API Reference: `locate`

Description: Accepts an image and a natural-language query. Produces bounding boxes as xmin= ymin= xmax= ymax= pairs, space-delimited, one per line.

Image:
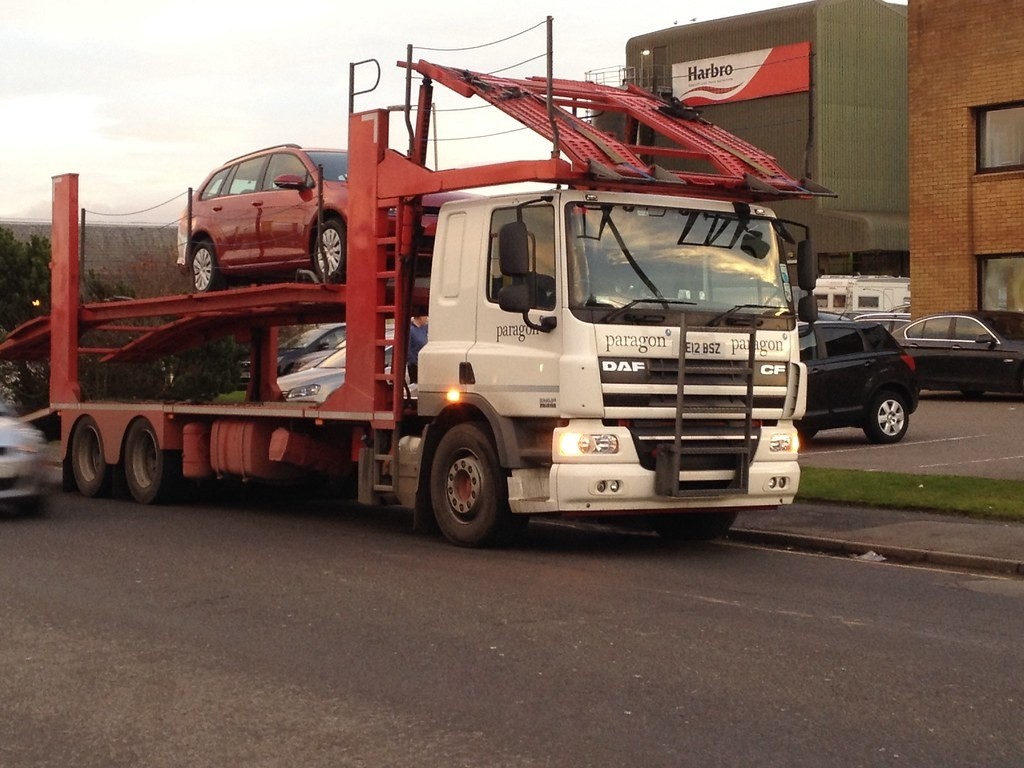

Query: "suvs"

xmin=796 ymin=321 xmax=923 ymax=447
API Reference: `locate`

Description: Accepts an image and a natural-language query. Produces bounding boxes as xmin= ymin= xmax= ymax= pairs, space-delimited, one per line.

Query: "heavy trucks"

xmin=1 ymin=14 xmax=839 ymax=552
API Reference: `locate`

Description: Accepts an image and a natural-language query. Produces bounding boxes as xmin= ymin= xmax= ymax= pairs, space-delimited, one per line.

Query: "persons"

xmin=407 ymin=316 xmax=429 ymax=384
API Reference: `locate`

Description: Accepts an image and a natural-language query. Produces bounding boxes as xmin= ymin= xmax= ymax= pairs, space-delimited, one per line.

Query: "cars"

xmin=888 ymin=310 xmax=1024 ymax=400
xmin=1 ymin=418 xmax=44 ymax=519
xmin=816 ymin=309 xmax=912 ymax=334
